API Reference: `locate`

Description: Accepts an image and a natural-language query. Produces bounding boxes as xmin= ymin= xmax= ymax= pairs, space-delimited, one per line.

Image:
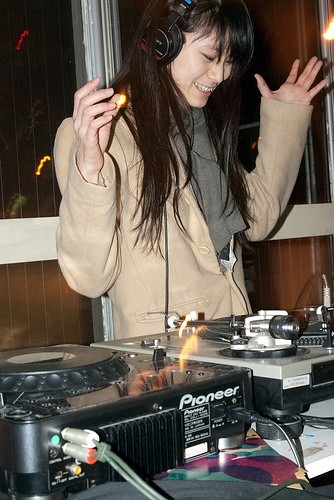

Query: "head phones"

xmin=140 ymin=0 xmax=200 ymax=68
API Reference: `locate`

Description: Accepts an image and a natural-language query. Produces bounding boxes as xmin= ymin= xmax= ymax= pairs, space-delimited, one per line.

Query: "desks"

xmin=250 ymin=398 xmax=334 ymax=479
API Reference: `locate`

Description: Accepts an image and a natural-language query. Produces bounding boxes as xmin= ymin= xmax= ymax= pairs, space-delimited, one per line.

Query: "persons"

xmin=52 ymin=0 xmax=331 ymax=337
